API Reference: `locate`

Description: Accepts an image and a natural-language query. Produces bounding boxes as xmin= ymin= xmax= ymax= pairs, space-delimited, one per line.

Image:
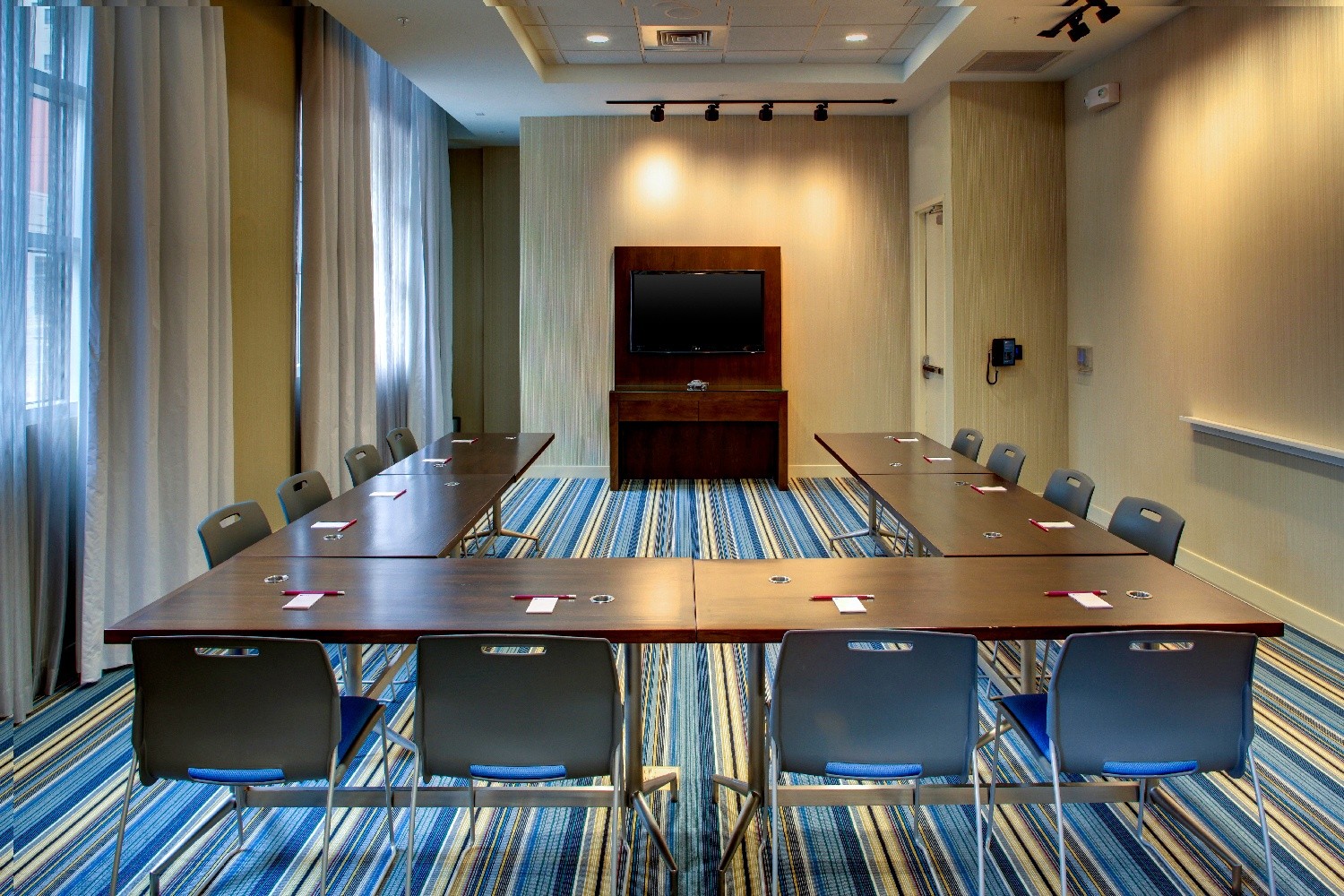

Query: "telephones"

xmin=992 ymin=338 xmax=1015 ymax=366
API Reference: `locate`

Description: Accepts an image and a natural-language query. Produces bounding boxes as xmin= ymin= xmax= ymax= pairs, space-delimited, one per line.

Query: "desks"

xmin=857 ymin=472 xmax=1150 ymax=558
xmin=237 ymin=475 xmax=542 ymax=558
xmin=103 ymin=549 xmax=694 ymax=896
xmin=691 ymin=555 xmax=1284 ymax=892
xmin=608 ymin=389 xmax=789 ymax=491
xmin=811 ymin=431 xmax=999 ymax=551
xmin=375 ymin=432 xmax=556 ymax=548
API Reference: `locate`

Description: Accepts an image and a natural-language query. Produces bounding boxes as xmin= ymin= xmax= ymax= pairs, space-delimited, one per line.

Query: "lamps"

xmin=1067 ymin=15 xmax=1090 ymax=43
xmin=650 ymin=103 xmax=665 ymax=122
xmin=1089 ymin=0 xmax=1120 ymax=23
xmin=814 ymin=102 xmax=828 ymax=121
xmin=759 ymin=103 xmax=773 ymax=121
xmin=705 ymin=104 xmax=719 ymax=121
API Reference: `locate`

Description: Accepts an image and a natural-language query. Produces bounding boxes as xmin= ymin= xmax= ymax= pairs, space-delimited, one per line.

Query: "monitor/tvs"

xmin=630 ymin=269 xmax=766 ymax=355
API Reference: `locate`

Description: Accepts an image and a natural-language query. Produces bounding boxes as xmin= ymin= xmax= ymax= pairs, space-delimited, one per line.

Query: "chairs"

xmin=109 ymin=426 xmax=1277 ymax=895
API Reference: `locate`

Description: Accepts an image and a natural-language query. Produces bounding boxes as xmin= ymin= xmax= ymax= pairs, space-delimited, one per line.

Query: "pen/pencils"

xmin=510 ymin=594 xmax=577 ymax=599
xmin=970 ymin=485 xmax=986 ymax=495
xmin=1028 ymin=519 xmax=1048 ymax=532
xmin=393 ymin=489 xmax=407 ymax=500
xmin=443 ymin=457 xmax=452 ymax=464
xmin=471 ymin=438 xmax=479 ymax=444
xmin=923 ymin=455 xmax=933 ymax=464
xmin=893 ymin=437 xmax=901 ymax=443
xmin=338 ymin=518 xmax=357 ymax=532
xmin=280 ymin=589 xmax=346 ymax=596
xmin=1043 ymin=590 xmax=1108 ymax=596
xmin=810 ymin=594 xmax=875 ymax=600
